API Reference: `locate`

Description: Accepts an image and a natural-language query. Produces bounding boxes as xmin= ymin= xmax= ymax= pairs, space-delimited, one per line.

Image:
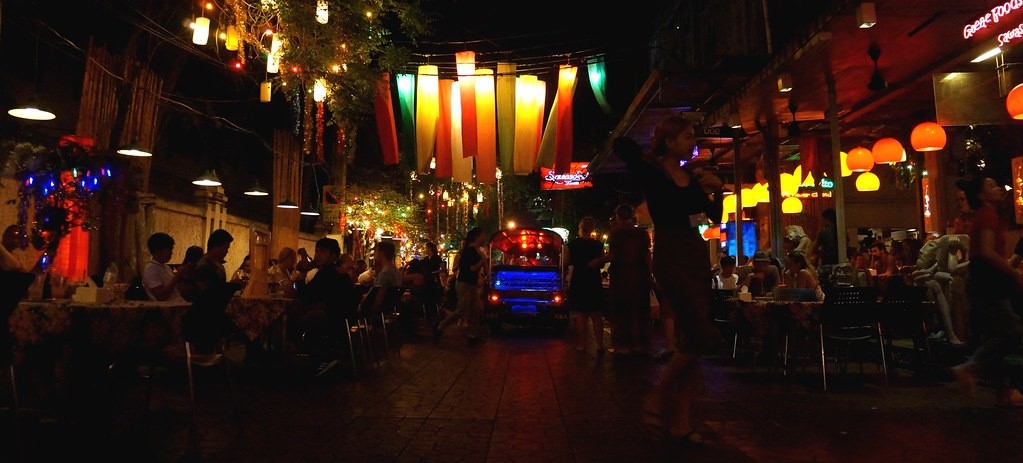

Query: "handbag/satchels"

xmin=648 ymin=288 xmax=662 ymax=322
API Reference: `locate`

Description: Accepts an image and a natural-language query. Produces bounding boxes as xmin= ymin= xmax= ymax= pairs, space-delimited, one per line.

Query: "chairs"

xmin=821 ymin=286 xmax=890 ymax=391
xmin=372 ymin=286 xmax=405 ymax=362
xmin=358 ymin=285 xmax=382 ymax=369
xmin=876 ymin=284 xmax=937 ymax=384
xmin=320 ymin=284 xmax=371 ymax=380
xmin=711 ymin=289 xmax=736 ymax=328
xmin=138 ymin=280 xmax=241 ymax=457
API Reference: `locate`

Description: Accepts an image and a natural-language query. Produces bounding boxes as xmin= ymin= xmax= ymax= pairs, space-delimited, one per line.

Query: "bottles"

xmin=103 ymin=262 xmax=119 ymax=289
xmin=268 ymin=276 xmax=285 ymax=296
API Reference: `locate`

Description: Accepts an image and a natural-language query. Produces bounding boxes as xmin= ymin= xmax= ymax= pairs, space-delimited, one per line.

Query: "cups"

xmin=766 ymin=290 xmax=773 ymax=301
xmin=28 ymin=274 xmax=45 ymax=300
xmin=50 ymin=274 xmax=69 ymax=301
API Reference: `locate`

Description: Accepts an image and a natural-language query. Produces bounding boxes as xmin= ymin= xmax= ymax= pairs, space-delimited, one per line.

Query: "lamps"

xmin=267 ymin=54 xmax=279 ymax=73
xmin=1005 ymin=83 xmax=1023 ymax=121
xmin=374 ymin=48 xmax=610 ymax=185
xmin=300 ymin=202 xmax=321 ymax=216
xmin=243 ymin=178 xmax=269 ymax=196
xmin=260 ymin=73 xmax=272 ymax=103
xmin=191 ymin=168 xmax=222 ymax=187
xmin=6 ymin=26 xmax=56 ymax=121
xmin=313 ymin=77 xmax=326 ymax=103
xmin=315 ymin=0 xmax=328 ymax=24
xmin=192 ymin=0 xmax=210 ymax=46
xmin=271 ymin=24 xmax=284 ymax=56
xmin=846 ymin=147 xmax=874 ymax=172
xmin=225 ymin=25 xmax=239 ymax=51
xmin=855 ymin=171 xmax=880 ymax=191
xmin=116 ymin=93 xmax=153 ymax=158
xmin=872 ymin=138 xmax=906 ymax=164
xmin=910 ymin=122 xmax=947 ymax=152
xmin=708 ymin=151 xmax=853 ymax=224
xmin=776 ymin=73 xmax=793 ymax=93
xmin=276 ymin=197 xmax=299 ymax=209
xmin=855 ymin=2 xmax=877 ymax=29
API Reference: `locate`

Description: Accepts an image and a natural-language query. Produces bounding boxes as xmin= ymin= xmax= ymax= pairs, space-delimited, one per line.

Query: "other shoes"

xmin=467 ymin=335 xmax=487 ymax=344
xmin=430 ymin=321 xmax=442 ymax=339
xmin=313 ymin=355 xmax=337 ymax=377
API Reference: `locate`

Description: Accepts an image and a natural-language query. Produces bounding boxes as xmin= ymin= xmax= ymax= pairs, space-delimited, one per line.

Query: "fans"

xmin=866 ymin=42 xmax=902 ymax=92
xmin=705 ymin=147 xmax=733 ymax=169
xmin=736 ymin=122 xmax=760 ymax=143
xmin=785 ymin=99 xmax=831 ymax=140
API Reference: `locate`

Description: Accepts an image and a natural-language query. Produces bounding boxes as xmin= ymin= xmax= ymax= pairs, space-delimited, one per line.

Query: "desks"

xmin=227 ymin=294 xmax=299 ymax=396
xmin=729 ymin=300 xmax=936 ymax=384
xmin=7 ymin=298 xmax=193 ymax=463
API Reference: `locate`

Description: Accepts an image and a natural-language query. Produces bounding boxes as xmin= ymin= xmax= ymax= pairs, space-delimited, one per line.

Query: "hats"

xmin=754 ymin=251 xmax=770 ymax=263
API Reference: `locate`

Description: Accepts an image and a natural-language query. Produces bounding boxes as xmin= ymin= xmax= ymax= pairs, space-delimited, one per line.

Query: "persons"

xmin=0 ymin=115 xmax=1023 ymax=463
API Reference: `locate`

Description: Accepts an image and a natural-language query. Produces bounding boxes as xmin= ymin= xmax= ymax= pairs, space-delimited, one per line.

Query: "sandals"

xmin=679 ymin=430 xmax=710 ymax=450
